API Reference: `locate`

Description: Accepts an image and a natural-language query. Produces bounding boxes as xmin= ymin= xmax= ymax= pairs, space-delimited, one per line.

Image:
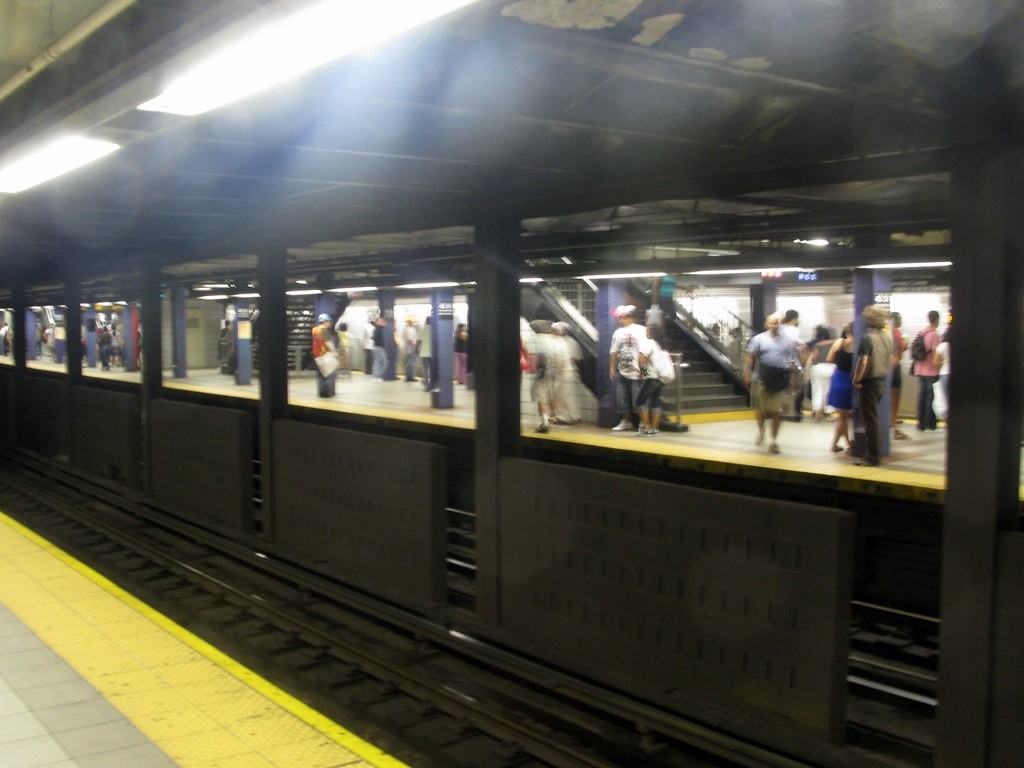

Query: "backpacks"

xmin=650 ymin=339 xmax=675 ymax=384
xmin=911 ymin=330 xmax=934 ymax=362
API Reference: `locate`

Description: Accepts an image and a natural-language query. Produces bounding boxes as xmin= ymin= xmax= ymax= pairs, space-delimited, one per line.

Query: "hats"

xmin=318 ymin=313 xmax=331 ymax=322
xmin=405 ymin=317 xmax=416 ymax=323
xmin=614 ymin=305 xmax=636 ymax=318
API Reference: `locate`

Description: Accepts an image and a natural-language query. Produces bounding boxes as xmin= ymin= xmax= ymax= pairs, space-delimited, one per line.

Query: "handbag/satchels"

xmin=315 ymin=352 xmax=339 ymax=377
xmin=759 ymin=365 xmax=793 ymax=392
xmin=530 ymin=353 xmax=545 ymax=380
xmin=646 ymin=303 xmax=663 ymax=326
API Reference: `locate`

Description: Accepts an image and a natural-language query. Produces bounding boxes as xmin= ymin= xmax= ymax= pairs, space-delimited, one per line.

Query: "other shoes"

xmin=633 ymin=428 xmax=658 ymax=439
xmin=833 ymin=447 xmax=843 ymax=452
xmin=612 ymin=419 xmax=633 ymax=431
xmin=854 ymin=462 xmax=879 ymax=467
xmin=407 ymin=378 xmax=418 ymax=381
xmin=537 ymin=424 xmax=548 ymax=433
xmin=895 ymin=430 xmax=911 ymax=441
xmin=917 ymin=424 xmax=944 ymax=433
xmin=756 ymin=436 xmax=763 ymax=445
xmin=770 ymin=445 xmax=778 ymax=454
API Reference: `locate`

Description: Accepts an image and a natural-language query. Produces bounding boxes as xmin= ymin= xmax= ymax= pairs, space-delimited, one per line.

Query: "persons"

xmin=518 ymin=274 xmax=951 ymax=467
xmin=312 ymin=310 xmax=469 ymax=398
xmin=0 ymin=319 xmax=142 ymax=372
xmin=219 ymin=320 xmax=229 ymax=339
